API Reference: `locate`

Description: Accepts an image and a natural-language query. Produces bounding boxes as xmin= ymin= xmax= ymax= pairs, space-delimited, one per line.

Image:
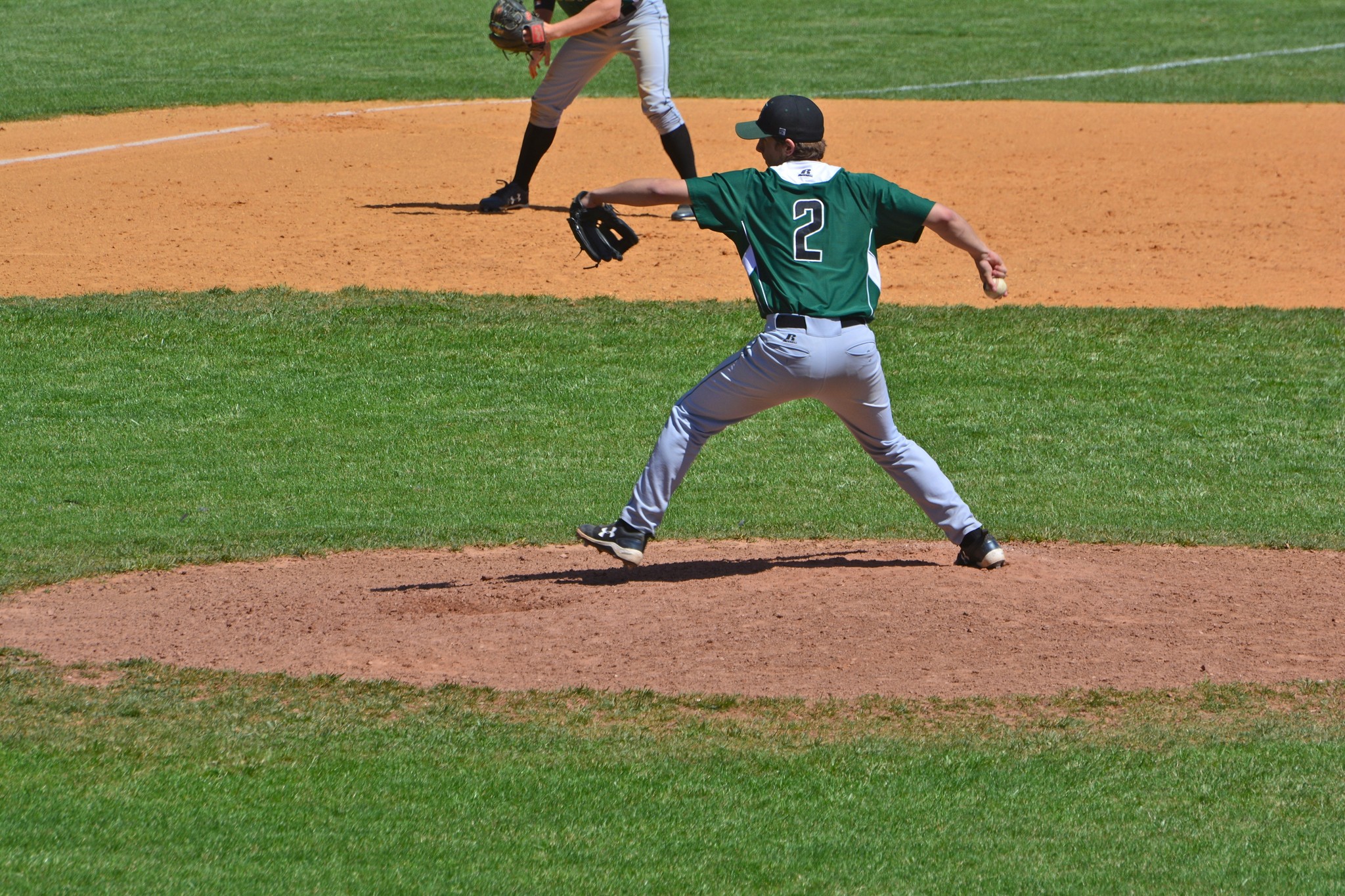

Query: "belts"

xmin=775 ymin=314 xmax=868 ymax=330
xmin=611 ymin=3 xmax=636 ymax=25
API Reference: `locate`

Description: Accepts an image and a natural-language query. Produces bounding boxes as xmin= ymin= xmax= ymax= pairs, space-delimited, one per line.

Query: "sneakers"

xmin=671 ymin=204 xmax=696 ymax=221
xmin=953 ymin=528 xmax=1006 ymax=571
xmin=574 ymin=518 xmax=649 ymax=570
xmin=478 ymin=179 xmax=530 ymax=214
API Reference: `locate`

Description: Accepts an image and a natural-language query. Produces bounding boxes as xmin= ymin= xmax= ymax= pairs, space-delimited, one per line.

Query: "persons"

xmin=477 ymin=0 xmax=695 ymax=221
xmin=575 ymin=94 xmax=1007 ymax=571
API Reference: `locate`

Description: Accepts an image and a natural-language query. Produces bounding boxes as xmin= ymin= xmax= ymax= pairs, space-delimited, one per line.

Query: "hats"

xmin=735 ymin=95 xmax=825 ymax=144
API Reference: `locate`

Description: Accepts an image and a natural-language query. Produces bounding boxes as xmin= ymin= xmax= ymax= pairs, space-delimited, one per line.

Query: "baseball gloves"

xmin=489 ymin=0 xmax=545 ymax=53
xmin=567 ymin=191 xmax=639 ymax=263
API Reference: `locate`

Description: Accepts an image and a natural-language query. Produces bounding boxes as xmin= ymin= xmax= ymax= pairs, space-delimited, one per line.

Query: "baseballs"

xmin=983 ymin=276 xmax=1007 ymax=299
